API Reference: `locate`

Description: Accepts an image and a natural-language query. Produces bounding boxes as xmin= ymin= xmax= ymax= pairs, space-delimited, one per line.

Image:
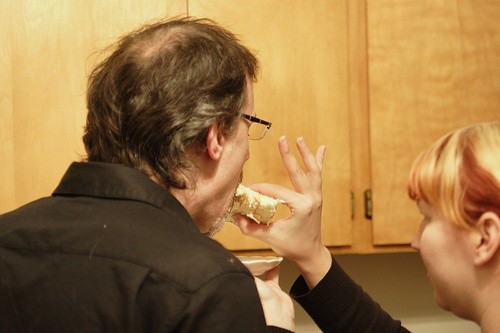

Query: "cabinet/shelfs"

xmin=1 ymin=1 xmax=500 ymax=258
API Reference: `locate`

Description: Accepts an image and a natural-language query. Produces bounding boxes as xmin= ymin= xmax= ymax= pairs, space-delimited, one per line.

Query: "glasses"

xmin=236 ymin=112 xmax=272 ymax=141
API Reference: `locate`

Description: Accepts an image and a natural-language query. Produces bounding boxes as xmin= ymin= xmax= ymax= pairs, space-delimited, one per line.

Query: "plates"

xmin=235 ymin=254 xmax=282 ymax=278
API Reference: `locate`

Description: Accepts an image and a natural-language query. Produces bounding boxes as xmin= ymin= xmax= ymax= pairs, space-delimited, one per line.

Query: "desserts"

xmin=224 ymin=184 xmax=283 ymax=226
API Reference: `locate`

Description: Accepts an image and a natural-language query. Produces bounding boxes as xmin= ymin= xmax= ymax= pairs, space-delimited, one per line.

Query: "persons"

xmin=249 ymin=121 xmax=500 ymax=333
xmin=0 ymin=16 xmax=273 ymax=333
